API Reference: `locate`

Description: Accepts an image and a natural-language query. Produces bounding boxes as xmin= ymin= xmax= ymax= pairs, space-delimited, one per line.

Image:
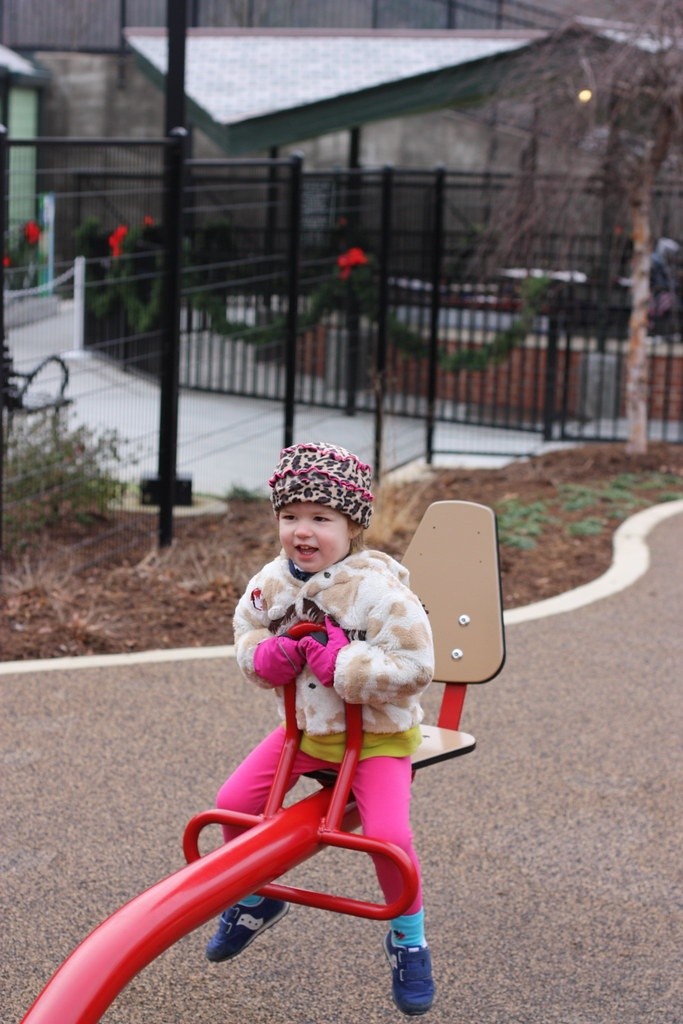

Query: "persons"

xmin=609 ymin=237 xmax=681 ymax=322
xmin=205 ymin=441 xmax=471 ymax=1017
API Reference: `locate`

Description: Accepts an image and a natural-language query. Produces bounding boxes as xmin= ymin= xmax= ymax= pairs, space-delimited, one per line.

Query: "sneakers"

xmin=206 ymin=894 xmax=289 ymax=963
xmin=383 ymin=930 xmax=435 ymax=1015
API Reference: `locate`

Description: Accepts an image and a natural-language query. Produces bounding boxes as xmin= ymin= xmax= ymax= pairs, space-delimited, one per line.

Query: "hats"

xmin=269 ymin=441 xmax=373 ymax=530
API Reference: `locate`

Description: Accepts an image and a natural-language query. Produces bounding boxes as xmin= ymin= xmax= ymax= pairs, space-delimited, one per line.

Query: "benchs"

xmin=0 ymin=331 xmax=72 ymax=449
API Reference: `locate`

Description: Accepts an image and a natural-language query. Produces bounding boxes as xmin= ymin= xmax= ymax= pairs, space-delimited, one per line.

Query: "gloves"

xmin=254 ymin=636 xmax=306 ymax=685
xmin=297 ymin=612 xmax=349 ymax=688
